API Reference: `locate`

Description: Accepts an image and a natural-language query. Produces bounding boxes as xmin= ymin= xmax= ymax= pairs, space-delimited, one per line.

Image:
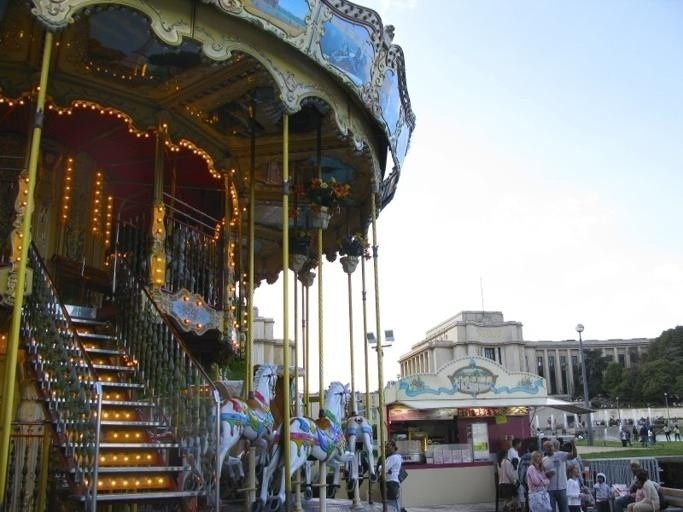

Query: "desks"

xmin=614 ymin=396 xmax=621 ymax=419
xmin=367 ymin=329 xmax=394 ymax=355
xmin=663 ymin=392 xmax=671 ymax=418
xmin=574 ymin=323 xmax=594 ymax=447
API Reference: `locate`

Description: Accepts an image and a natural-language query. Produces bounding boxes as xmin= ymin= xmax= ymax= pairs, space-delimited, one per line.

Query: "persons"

xmin=378 ymin=440 xmax=402 ymax=500
xmin=494 ymin=414 xmax=683 ymax=512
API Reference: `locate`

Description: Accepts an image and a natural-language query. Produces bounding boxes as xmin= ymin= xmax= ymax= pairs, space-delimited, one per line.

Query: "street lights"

xmin=613 ymin=484 xmax=627 ymax=495
xmin=398 ymin=467 xmax=408 ymax=482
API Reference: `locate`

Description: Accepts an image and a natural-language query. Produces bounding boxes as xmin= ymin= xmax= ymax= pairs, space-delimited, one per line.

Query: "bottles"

xmin=597 ymin=473 xmax=606 ymax=482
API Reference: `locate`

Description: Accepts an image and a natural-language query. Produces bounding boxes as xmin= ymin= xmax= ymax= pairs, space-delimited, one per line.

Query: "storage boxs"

xmin=656 ymin=487 xmax=683 ymax=512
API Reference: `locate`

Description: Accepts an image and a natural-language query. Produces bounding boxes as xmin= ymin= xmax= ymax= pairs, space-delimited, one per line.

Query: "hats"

xmin=591 ymin=415 xmax=682 ymax=426
xmin=571 ymin=427 xmax=591 ymax=441
